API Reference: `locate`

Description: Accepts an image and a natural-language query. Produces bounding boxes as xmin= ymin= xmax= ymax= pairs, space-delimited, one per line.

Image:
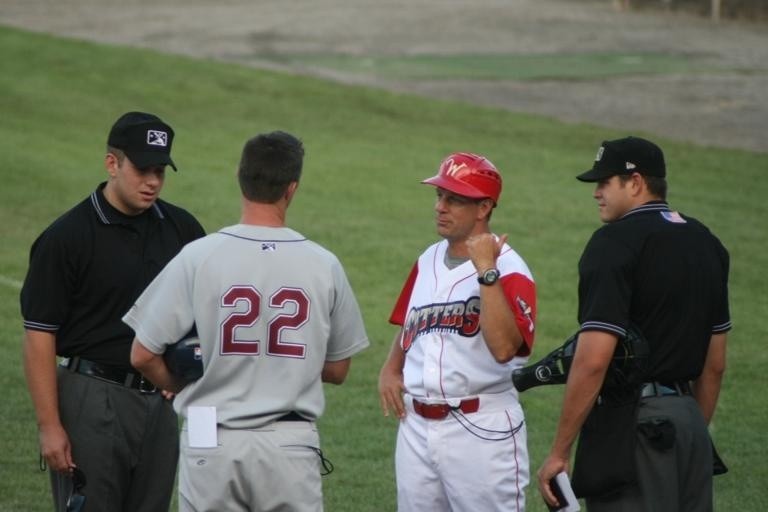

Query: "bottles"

xmin=577 ymin=136 xmax=664 ymax=182
xmin=109 ymin=111 xmax=178 ymax=173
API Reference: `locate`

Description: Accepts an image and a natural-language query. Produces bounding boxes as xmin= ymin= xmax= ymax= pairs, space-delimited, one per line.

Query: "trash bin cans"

xmin=476 ymin=268 xmax=501 ymax=285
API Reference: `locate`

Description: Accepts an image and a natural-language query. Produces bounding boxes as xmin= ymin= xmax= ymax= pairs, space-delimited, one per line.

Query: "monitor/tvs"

xmin=542 ymin=472 xmax=582 ymax=512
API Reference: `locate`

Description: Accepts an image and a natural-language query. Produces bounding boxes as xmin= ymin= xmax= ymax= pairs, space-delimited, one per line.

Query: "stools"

xmin=640 ymin=379 xmax=691 ymax=399
xmin=413 ymin=398 xmax=479 ymax=419
xmin=58 ymin=356 xmax=159 ymax=395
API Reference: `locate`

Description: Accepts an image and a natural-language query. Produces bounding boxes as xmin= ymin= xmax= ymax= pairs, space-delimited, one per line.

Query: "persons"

xmin=534 ymin=132 xmax=735 ymax=512
xmin=118 ymin=128 xmax=371 ymax=511
xmin=18 ymin=109 xmax=207 ymax=512
xmin=376 ymin=150 xmax=541 ymax=512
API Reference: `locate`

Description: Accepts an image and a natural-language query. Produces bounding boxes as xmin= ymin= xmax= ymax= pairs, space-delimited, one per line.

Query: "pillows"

xmin=420 ymin=152 xmax=502 ymax=207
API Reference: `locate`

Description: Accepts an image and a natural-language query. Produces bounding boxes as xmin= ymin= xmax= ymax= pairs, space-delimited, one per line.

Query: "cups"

xmin=65 ymin=466 xmax=87 ymax=511
